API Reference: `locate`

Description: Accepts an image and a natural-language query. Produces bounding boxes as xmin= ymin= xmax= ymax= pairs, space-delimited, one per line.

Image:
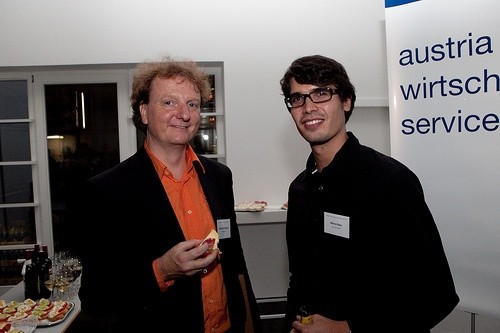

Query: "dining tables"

xmin=0 ymin=276 xmax=85 ymax=333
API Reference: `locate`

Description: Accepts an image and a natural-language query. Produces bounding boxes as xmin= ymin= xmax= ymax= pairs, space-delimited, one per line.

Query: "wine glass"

xmin=44 ymin=250 xmax=83 ymax=301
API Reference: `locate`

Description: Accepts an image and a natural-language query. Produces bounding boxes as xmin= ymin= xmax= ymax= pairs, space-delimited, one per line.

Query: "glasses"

xmin=284 ymin=86 xmax=338 ymax=109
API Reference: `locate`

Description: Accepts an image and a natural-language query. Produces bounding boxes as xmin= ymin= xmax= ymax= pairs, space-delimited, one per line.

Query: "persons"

xmin=71 ymin=62 xmax=264 ymax=333
xmin=281 ymin=55 xmax=460 ymax=333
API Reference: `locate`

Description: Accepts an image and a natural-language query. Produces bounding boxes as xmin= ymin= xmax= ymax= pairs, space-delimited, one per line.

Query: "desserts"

xmin=200 ymin=229 xmax=219 ymax=254
xmin=0 ymin=298 xmax=69 ymax=333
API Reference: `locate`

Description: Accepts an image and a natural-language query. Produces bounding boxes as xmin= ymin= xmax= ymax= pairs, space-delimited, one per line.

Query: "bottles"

xmin=300 ymin=305 xmax=313 ymax=325
xmin=24 ymin=245 xmax=52 ymax=301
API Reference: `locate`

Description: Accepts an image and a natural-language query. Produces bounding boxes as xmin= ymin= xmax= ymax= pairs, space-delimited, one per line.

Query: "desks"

xmin=235 ymin=208 xmax=294 ymax=318
